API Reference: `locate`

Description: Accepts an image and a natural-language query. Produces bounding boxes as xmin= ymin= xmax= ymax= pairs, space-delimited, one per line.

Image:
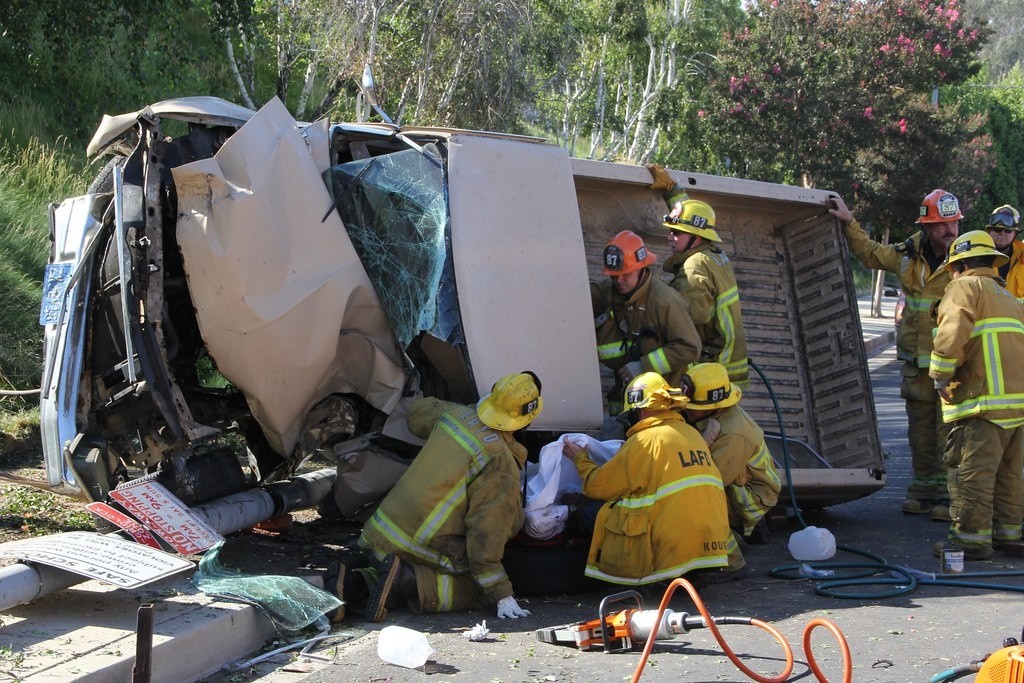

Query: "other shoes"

xmin=696 ymin=566 xmax=746 ymax=584
xmin=669 ymin=568 xmax=702 ymax=596
xmin=933 ymin=541 xmax=992 ymax=561
xmin=599 ymin=583 xmax=629 ymax=601
xmin=901 ymin=497 xmax=934 ymax=513
xmin=991 ymin=537 xmax=1022 ymax=549
xmin=931 ymin=506 xmax=953 ymax=521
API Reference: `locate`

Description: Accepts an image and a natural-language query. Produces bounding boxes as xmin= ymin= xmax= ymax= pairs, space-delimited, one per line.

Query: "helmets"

xmin=478 ymin=371 xmax=542 ymax=432
xmin=943 ymin=230 xmax=1010 ymax=272
xmin=985 ymin=204 xmax=1024 ymax=232
xmin=620 ymin=372 xmax=690 ymax=416
xmin=663 ymin=197 xmax=724 ymax=245
xmin=675 ymin=362 xmax=742 ymax=409
xmin=914 ymin=188 xmax=964 ymax=224
xmin=601 ymin=230 xmax=657 ymax=276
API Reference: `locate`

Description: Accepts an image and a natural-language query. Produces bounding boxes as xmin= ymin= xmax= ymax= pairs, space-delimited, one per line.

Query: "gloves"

xmin=646 ymin=163 xmax=676 ymax=193
xmin=496 ymin=595 xmax=531 ymax=619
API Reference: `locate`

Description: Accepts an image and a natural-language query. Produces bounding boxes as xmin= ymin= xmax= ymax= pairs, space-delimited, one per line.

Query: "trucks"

xmin=37 ymin=96 xmax=891 ymax=538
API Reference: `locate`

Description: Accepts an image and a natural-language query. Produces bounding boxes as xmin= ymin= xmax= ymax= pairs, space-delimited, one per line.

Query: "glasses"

xmin=988 ymin=213 xmax=1017 ymax=226
xmin=670 ymin=228 xmax=689 ymax=235
xmin=992 ymin=228 xmax=1014 ymax=233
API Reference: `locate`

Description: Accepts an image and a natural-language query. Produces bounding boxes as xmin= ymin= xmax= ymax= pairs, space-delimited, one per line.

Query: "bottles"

xmin=799 ymin=563 xmax=834 ymax=576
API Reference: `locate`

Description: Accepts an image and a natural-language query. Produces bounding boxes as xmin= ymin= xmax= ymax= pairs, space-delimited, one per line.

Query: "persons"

xmin=563 ymin=372 xmax=731 ymax=598
xmin=928 ymin=202 xmax=1024 ymax=558
xmin=677 ymin=362 xmax=781 ymax=546
xmin=829 ymin=189 xmax=965 ymax=521
xmin=645 ymin=164 xmax=750 ymax=391
xmin=589 ymin=230 xmax=702 ymax=416
xmin=322 ymin=370 xmax=544 ymax=623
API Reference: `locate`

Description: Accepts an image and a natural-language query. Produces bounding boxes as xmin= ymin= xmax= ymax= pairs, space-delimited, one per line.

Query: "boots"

xmin=322 ymin=560 xmax=371 ymax=624
xmin=366 ymin=553 xmax=417 ymax=623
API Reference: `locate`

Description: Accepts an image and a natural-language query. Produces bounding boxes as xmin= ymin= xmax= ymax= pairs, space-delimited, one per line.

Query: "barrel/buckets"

xmin=378 ymin=626 xmax=434 ymax=669
xmin=941 ymin=549 xmax=964 ymax=574
xmin=785 ymin=525 xmax=836 ymax=561
